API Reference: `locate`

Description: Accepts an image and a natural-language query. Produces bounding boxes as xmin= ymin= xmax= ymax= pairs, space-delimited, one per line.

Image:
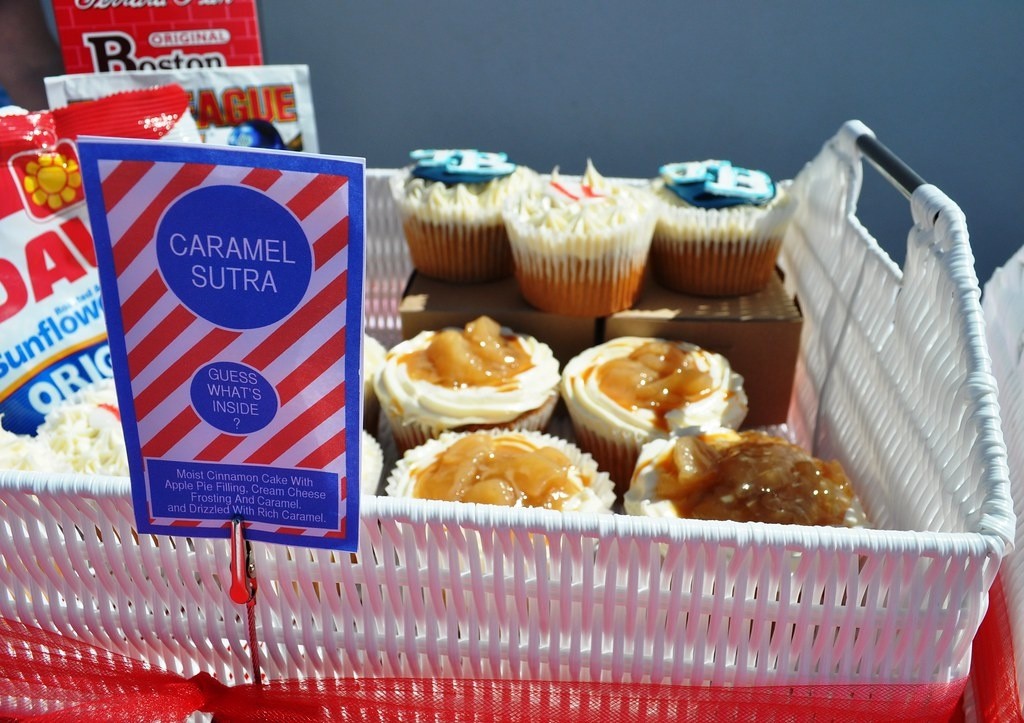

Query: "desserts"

xmin=389 ymin=148 xmax=802 ymax=318
xmin=365 ymin=316 xmax=868 ymax=535
xmin=0 ymin=379 xmax=131 ymax=483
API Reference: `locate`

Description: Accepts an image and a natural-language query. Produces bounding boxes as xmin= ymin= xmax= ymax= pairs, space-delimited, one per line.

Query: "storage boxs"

xmin=396 ymin=268 xmax=805 ymax=428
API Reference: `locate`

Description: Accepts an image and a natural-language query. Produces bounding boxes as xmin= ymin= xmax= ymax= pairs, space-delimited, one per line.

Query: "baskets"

xmin=0 ymin=122 xmax=1017 ymax=723
